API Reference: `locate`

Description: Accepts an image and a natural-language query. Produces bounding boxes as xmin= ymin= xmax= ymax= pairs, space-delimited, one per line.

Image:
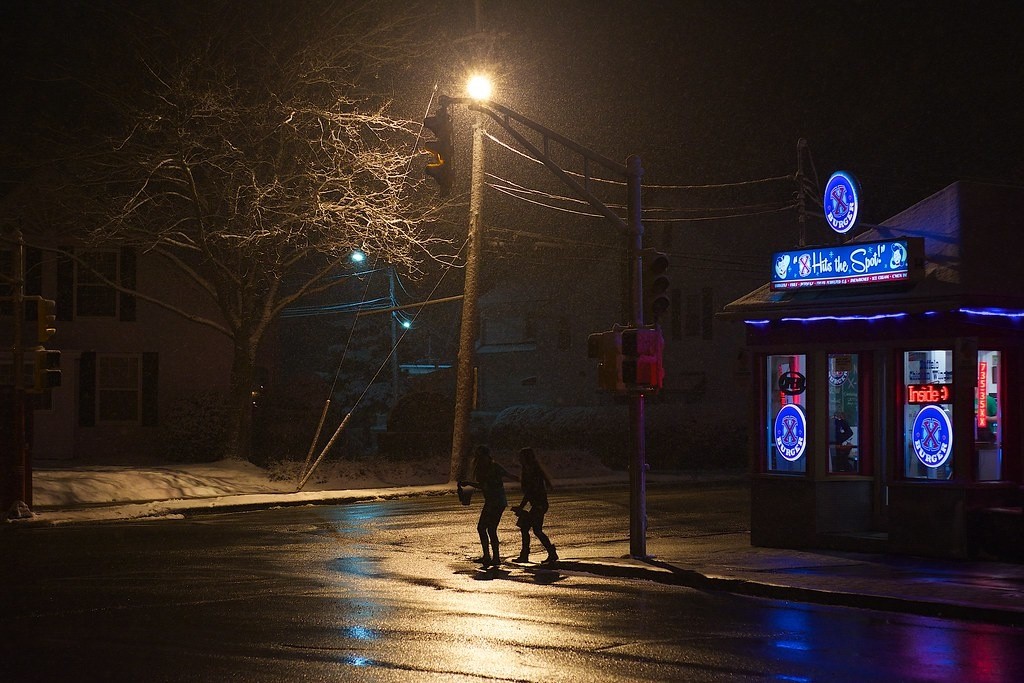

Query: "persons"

xmin=828 ymin=406 xmax=853 ymax=472
xmin=974 ymin=387 xmax=997 ymax=440
xmin=512 ymin=448 xmax=558 ymax=564
xmin=459 ymin=445 xmax=520 ymax=565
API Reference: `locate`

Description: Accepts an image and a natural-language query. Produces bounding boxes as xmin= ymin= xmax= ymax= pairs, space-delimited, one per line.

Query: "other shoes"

xmin=512 ymin=552 xmax=528 ymax=562
xmin=473 ymin=555 xmax=491 ymax=563
xmin=541 ymin=544 xmax=558 ymax=564
xmin=484 ymin=558 xmax=501 ymax=565
